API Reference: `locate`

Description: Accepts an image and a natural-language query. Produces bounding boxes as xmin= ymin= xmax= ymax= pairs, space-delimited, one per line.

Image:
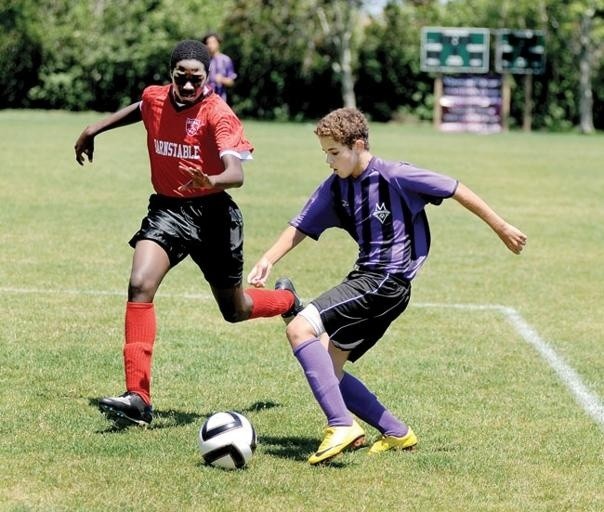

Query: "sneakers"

xmin=97 ymin=390 xmax=154 ymax=429
xmin=367 ymin=423 xmax=418 ymax=457
xmin=308 ymin=419 xmax=367 ymax=465
xmin=274 ymin=276 xmax=305 ymax=326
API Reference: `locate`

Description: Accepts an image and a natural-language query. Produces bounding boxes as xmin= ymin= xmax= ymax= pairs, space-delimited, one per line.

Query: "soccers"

xmin=200 ymin=412 xmax=257 ymax=467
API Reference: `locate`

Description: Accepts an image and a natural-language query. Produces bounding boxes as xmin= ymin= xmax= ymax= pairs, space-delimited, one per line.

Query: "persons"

xmin=197 ymin=32 xmax=236 ymax=104
xmin=72 ymin=40 xmax=306 ymax=430
xmin=245 ymin=107 xmax=528 ymax=466
